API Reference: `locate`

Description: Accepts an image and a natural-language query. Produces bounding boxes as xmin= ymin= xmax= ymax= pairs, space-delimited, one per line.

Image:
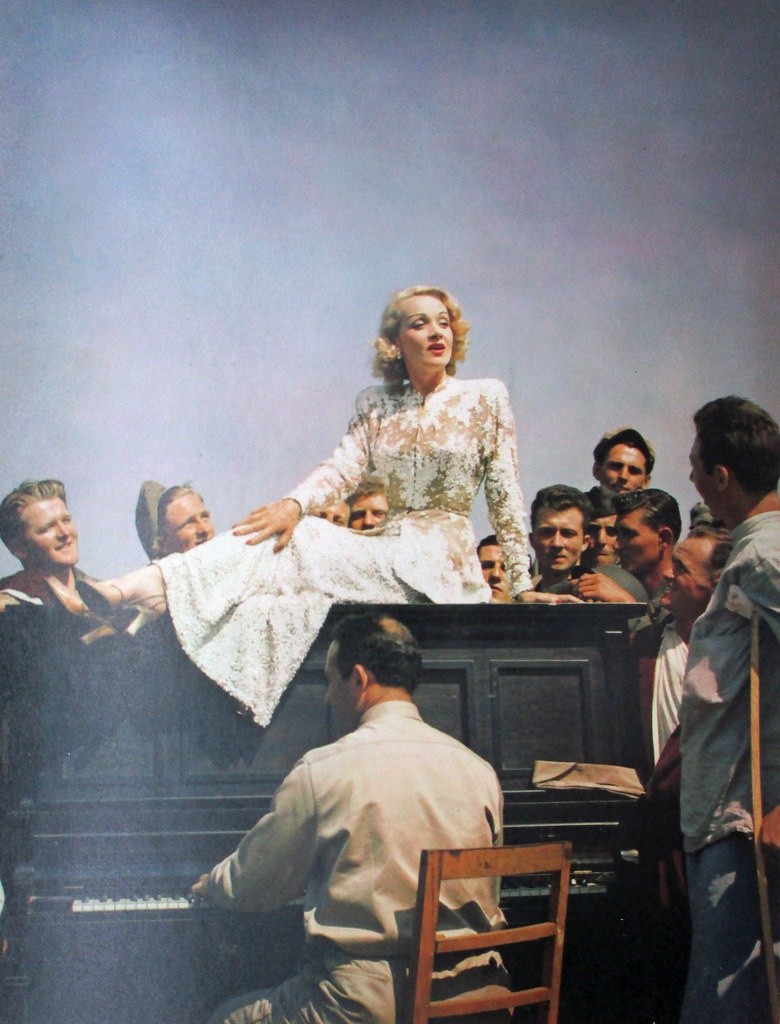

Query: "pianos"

xmin=0 ymin=599 xmax=657 ymax=1024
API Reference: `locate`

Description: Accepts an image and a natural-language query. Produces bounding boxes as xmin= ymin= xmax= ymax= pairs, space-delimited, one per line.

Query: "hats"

xmin=135 ymin=479 xmax=168 ymax=559
xmin=593 ymin=424 xmax=655 ymax=475
xmin=689 ymin=501 xmax=714 ymax=529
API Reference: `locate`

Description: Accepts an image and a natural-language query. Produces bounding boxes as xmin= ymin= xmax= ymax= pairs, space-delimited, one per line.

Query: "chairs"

xmin=410 ymin=842 xmax=575 ymax=1024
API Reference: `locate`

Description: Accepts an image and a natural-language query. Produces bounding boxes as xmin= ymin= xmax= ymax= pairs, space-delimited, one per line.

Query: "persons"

xmin=676 ymin=396 xmax=780 ymax=1024
xmin=192 ymin=611 xmax=507 ymax=1024
xmin=618 ymin=503 xmax=734 ymax=1024
xmin=1 ymin=429 xmax=720 ymax=615
xmin=44 ymin=285 xmax=585 ymax=647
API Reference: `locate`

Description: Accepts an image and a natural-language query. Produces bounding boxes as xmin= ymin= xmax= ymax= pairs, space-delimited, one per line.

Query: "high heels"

xmin=45 ymin=575 xmax=120 ymax=646
xmin=74 ymin=574 xmax=167 ymax=640
